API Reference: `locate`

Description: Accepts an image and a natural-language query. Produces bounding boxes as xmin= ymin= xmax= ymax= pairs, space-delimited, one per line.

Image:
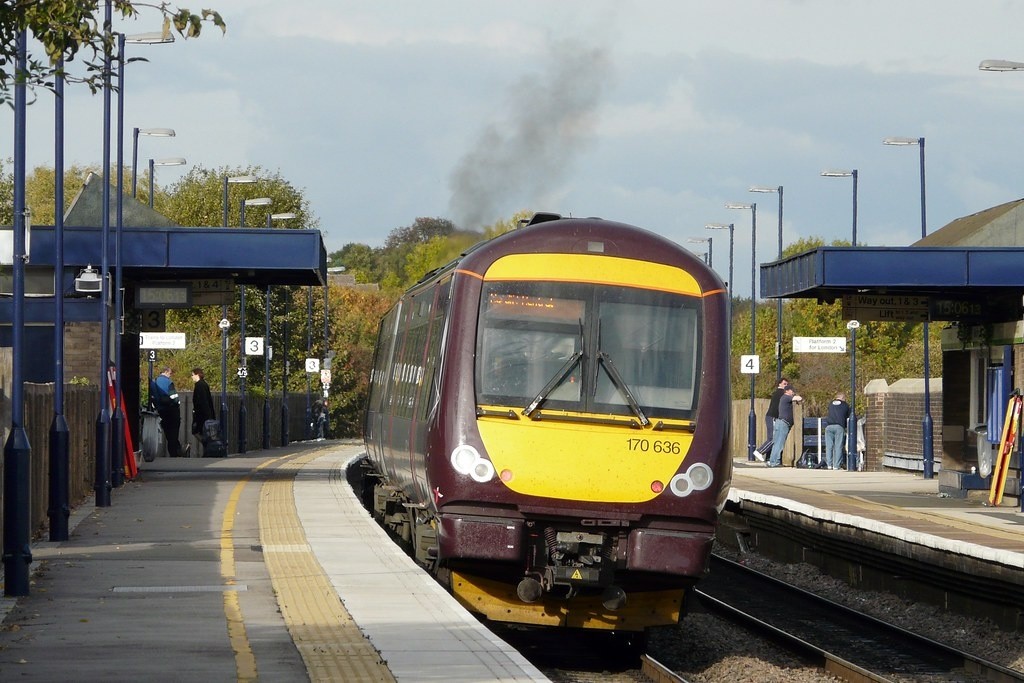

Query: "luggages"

xmin=204 ymin=420 xmax=226 ymax=457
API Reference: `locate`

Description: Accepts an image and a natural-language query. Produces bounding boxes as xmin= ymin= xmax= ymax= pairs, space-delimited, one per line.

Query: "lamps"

xmin=75 ymin=263 xmax=102 ymax=292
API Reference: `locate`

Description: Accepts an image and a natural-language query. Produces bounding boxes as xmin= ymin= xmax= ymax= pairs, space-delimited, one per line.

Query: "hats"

xmin=784 ymin=386 xmax=796 ymax=392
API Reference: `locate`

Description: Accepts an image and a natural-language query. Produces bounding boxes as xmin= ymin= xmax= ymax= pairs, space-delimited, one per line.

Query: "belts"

xmin=780 ymin=419 xmax=790 ymax=427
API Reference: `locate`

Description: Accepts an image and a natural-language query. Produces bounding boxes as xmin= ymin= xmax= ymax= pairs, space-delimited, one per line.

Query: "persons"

xmin=824 ymin=392 xmax=852 ymax=470
xmin=752 ymin=378 xmax=802 ymax=469
xmin=191 ymin=368 xmax=216 ymax=457
xmin=149 ymin=366 xmax=185 ymax=458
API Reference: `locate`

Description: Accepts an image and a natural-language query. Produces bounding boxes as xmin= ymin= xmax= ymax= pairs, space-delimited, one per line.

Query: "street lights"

xmin=111 ymin=32 xmax=177 ymax=487
xmin=220 ymin=176 xmax=259 ymax=458
xmin=238 ymin=198 xmax=273 ymax=450
xmin=881 ymin=136 xmax=934 ymax=478
xmin=305 ymin=256 xmax=333 ymax=438
xmin=748 ymin=184 xmax=782 ymax=385
xmin=131 ymin=127 xmax=176 ymax=200
xmin=321 ymin=266 xmax=347 ymax=439
xmin=702 ymin=223 xmax=734 ymax=357
xmin=686 ymin=236 xmax=712 ymax=268
xmin=262 ymin=211 xmax=296 ymax=449
xmin=819 ymin=168 xmax=857 ymax=471
xmin=148 ymin=156 xmax=186 ymax=412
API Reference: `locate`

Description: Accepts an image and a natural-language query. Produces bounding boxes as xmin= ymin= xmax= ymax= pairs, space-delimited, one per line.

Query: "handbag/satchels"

xmin=159 ymin=399 xmax=179 ymax=412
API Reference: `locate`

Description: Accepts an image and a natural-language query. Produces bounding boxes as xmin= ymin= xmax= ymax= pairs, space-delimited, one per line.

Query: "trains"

xmin=361 ymin=213 xmax=734 ymax=658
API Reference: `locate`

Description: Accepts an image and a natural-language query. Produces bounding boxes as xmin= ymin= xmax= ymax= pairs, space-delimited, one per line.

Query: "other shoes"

xmin=833 ymin=467 xmax=844 ymax=470
xmin=828 ymin=466 xmax=832 ymax=470
xmin=752 ymin=450 xmax=765 ymax=461
